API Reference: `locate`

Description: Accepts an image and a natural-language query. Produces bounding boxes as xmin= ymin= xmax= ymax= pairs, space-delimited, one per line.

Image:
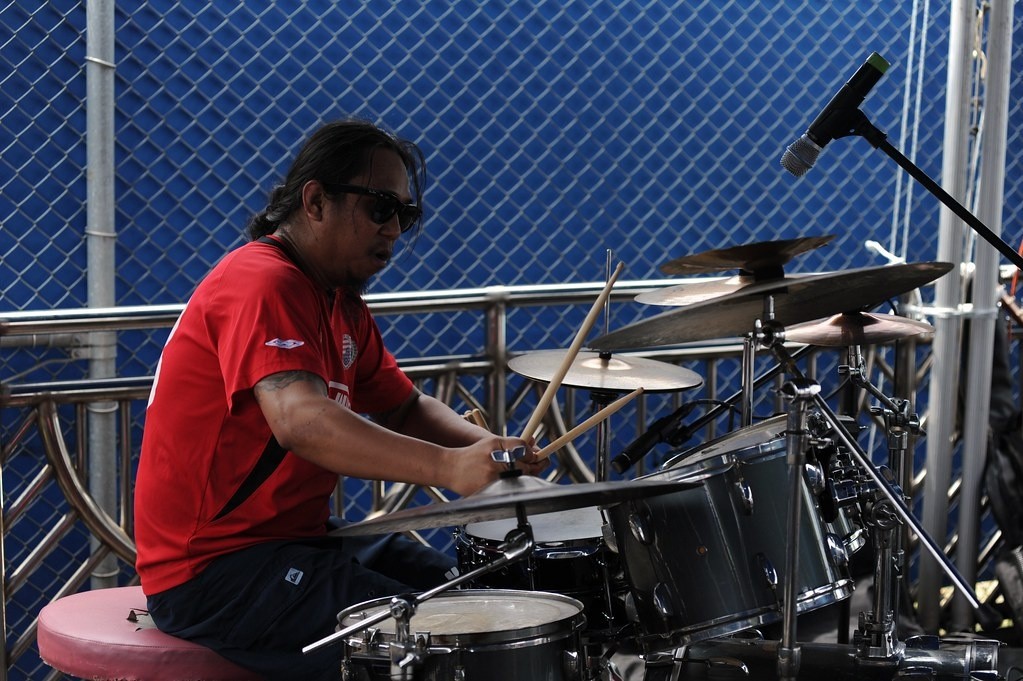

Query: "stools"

xmin=36 ymin=584 xmax=272 ymax=681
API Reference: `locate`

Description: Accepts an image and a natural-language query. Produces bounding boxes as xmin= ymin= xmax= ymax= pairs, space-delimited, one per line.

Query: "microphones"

xmin=611 ymin=400 xmax=697 ymax=474
xmin=781 ymin=51 xmax=891 ymax=177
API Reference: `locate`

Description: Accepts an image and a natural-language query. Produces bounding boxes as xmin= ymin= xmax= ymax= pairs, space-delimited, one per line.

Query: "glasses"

xmin=324 ymin=184 xmax=423 ymax=233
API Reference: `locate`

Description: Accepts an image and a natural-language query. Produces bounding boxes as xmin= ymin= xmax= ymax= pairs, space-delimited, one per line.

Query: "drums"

xmin=642 ymin=631 xmax=1004 ymax=681
xmin=333 ymin=585 xmax=589 ymax=680
xmin=452 ymin=486 xmax=624 ymax=650
xmin=602 ymin=411 xmax=869 ymax=650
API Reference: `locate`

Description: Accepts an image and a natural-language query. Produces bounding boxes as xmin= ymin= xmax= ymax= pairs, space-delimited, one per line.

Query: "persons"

xmin=133 ymin=121 xmax=550 ymax=681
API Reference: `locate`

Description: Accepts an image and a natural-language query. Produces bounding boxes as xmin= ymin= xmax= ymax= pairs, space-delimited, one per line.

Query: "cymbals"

xmin=324 ymin=471 xmax=706 ymax=539
xmin=583 ymin=234 xmax=955 ymax=360
xmin=506 ymin=347 xmax=707 ymax=391
